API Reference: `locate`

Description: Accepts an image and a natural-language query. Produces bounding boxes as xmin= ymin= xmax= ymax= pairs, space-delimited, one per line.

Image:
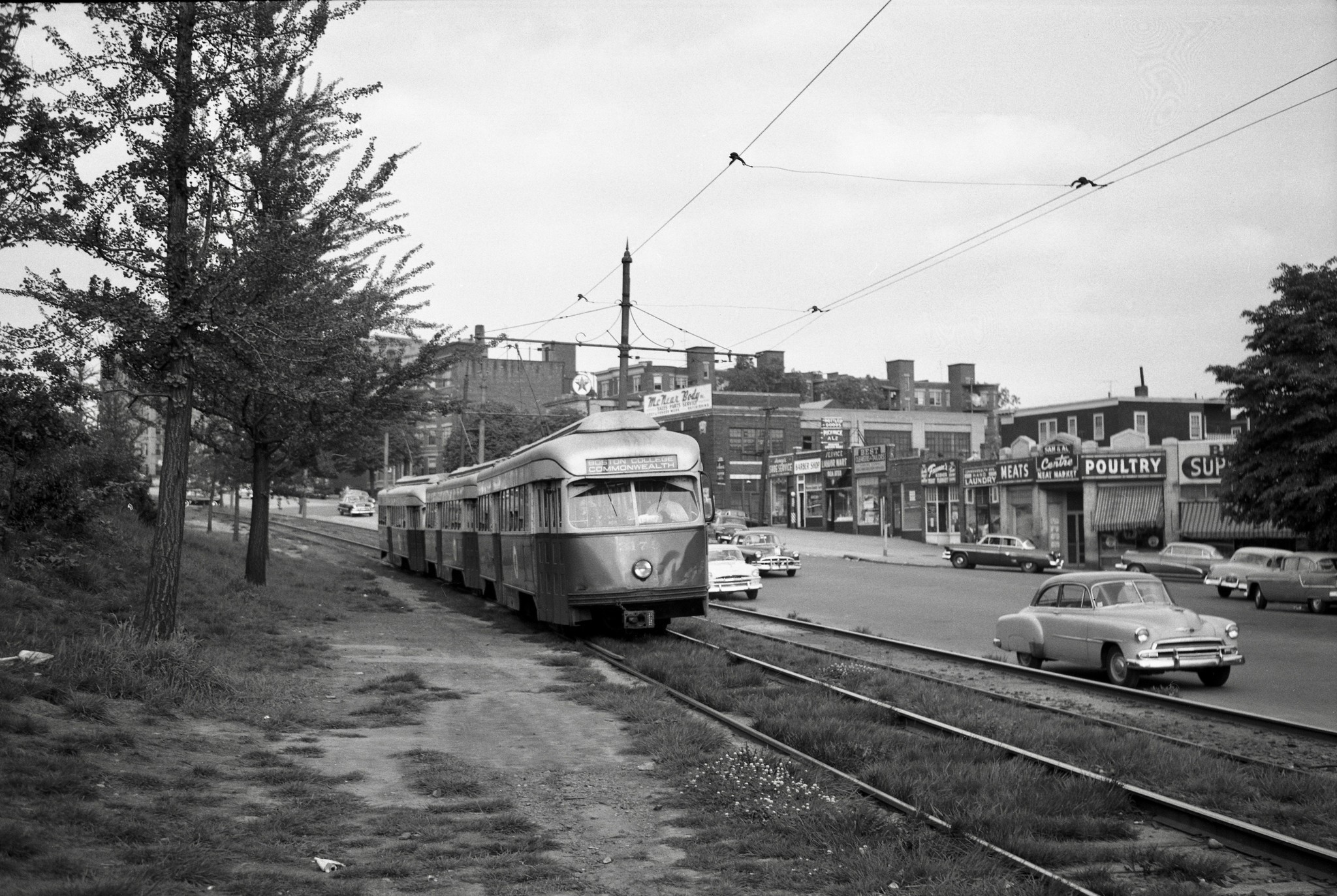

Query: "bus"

xmin=376 ymin=408 xmax=716 ymax=637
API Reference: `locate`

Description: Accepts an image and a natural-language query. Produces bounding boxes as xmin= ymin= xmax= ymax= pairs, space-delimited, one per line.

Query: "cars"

xmin=337 ymin=494 xmax=376 ymax=517
xmin=186 ymin=488 xmax=221 ymax=509
xmin=238 ymin=487 xmax=253 ymax=500
xmin=704 ymin=507 xmax=752 ymax=545
xmin=1203 ymin=545 xmax=1294 ymax=600
xmin=1245 ymin=551 xmax=1337 ymax=614
xmin=941 ymin=531 xmax=1065 ymax=573
xmin=706 ymin=543 xmax=763 ymax=600
xmin=1115 ymin=541 xmax=1228 ymax=580
xmin=992 ymin=569 xmax=1245 ymax=690
xmin=728 ymin=529 xmax=802 ymax=577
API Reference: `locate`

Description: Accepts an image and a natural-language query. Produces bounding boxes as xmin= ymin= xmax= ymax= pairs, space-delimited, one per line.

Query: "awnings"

xmin=1179 ymin=503 xmax=1311 ymax=539
xmin=1093 ymin=481 xmax=1164 ymax=532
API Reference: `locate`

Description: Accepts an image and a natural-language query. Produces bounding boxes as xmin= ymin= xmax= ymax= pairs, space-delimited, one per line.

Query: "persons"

xmin=645 ymin=487 xmax=688 ymax=523
